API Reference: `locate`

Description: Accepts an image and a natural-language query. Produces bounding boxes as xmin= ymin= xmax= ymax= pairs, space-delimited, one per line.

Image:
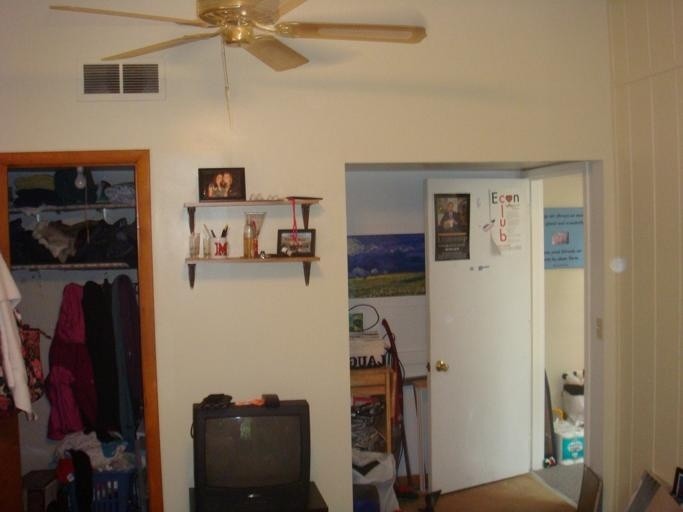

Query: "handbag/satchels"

xmin=0 ymin=312 xmax=51 ymax=417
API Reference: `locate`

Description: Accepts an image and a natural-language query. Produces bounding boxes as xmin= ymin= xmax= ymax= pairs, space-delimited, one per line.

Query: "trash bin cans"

xmin=563 ymin=383 xmax=584 ymax=426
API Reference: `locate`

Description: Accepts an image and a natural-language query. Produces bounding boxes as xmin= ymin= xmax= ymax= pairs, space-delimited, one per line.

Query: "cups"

xmin=210 ymin=238 xmax=227 ymax=259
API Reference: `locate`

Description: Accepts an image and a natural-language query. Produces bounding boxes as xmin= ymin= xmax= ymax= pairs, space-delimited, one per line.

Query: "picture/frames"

xmin=197 ymin=167 xmax=247 ymax=203
xmin=277 ymin=228 xmax=316 ymax=257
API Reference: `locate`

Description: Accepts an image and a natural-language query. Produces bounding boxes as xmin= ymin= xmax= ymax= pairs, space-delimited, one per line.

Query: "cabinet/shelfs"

xmin=182 ymin=197 xmax=322 ymax=287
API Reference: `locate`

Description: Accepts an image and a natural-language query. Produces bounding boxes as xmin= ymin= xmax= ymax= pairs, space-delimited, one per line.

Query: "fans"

xmin=48 ymin=0 xmax=428 ymax=73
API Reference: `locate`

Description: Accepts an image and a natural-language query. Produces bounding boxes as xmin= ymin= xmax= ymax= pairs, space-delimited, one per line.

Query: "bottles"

xmin=243 ymin=215 xmax=253 ymax=258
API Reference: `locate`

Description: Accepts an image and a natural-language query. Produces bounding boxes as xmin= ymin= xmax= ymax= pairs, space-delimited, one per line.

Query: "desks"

xmin=351 ymin=365 xmax=393 ymax=454
xmin=188 ymin=480 xmax=329 ymax=512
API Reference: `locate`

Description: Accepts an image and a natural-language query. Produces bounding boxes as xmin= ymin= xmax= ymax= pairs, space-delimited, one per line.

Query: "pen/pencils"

xmin=204 ymin=224 xmax=228 ymax=238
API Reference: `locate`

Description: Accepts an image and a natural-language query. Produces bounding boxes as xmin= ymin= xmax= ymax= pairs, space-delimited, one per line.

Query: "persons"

xmin=220 ymin=172 xmax=241 ymax=199
xmin=438 ymin=201 xmax=462 ymax=231
xmin=207 ymin=173 xmax=224 ymax=198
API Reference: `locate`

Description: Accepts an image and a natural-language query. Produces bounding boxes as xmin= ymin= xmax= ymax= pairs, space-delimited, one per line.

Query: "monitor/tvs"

xmin=192 ymin=399 xmax=312 ymax=505
